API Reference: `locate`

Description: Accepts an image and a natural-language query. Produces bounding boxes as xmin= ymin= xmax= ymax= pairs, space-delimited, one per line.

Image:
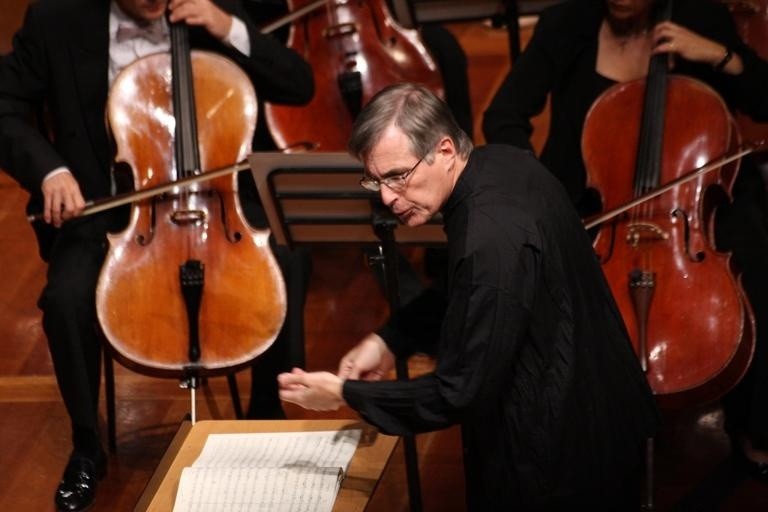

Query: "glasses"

xmin=360 ymin=152 xmax=432 ymax=191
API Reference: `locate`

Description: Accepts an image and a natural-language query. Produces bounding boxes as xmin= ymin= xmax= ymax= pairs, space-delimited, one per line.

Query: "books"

xmin=171 ymin=426 xmax=364 ymax=511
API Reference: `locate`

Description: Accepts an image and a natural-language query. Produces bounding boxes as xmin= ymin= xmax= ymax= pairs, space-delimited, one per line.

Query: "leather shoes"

xmin=54 ymin=444 xmax=107 ymax=512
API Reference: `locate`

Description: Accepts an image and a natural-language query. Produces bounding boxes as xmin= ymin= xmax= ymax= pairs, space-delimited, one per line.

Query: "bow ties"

xmin=116 ymin=21 xmax=163 ymax=44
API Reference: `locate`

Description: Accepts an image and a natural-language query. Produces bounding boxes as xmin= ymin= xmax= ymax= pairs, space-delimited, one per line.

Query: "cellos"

xmin=95 ymin=1 xmax=288 ymax=378
xmin=263 ymin=0 xmax=445 ymax=155
xmin=582 ymin=0 xmax=757 ymax=408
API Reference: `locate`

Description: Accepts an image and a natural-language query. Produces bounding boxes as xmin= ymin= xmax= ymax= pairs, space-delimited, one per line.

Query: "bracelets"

xmin=711 ymin=45 xmax=735 ymax=74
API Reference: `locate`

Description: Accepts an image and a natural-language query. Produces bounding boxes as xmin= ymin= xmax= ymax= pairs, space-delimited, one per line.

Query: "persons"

xmin=1 ymin=0 xmax=317 ymax=512
xmin=275 ymin=80 xmax=687 ymax=511
xmin=479 ymin=2 xmax=767 ymax=485
